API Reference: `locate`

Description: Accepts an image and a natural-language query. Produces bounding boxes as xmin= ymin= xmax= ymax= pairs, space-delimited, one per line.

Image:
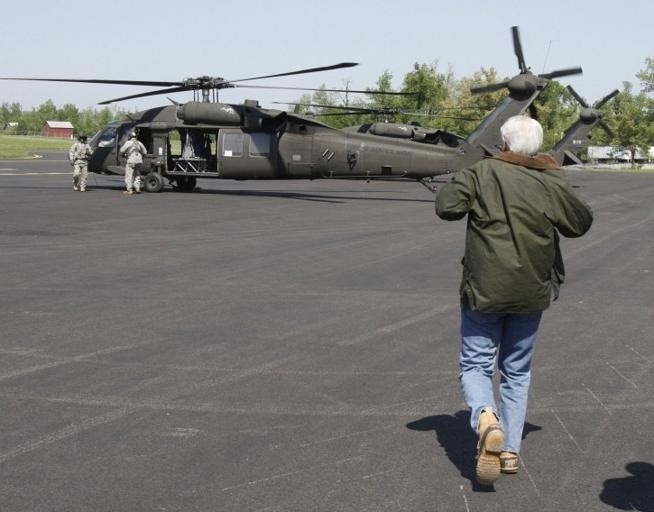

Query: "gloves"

xmin=70 ymin=161 xmax=74 ymax=166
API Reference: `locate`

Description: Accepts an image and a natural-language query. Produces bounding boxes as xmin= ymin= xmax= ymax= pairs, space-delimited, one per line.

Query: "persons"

xmin=68 ymin=134 xmax=95 ymax=192
xmin=433 ymin=114 xmax=594 ymax=488
xmin=119 ymin=130 xmax=148 ymax=196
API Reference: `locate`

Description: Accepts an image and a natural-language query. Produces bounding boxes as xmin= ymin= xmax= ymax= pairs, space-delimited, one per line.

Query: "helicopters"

xmin=0 ymin=25 xmax=619 ymax=193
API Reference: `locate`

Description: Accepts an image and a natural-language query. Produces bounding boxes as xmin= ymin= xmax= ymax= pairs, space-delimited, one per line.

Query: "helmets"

xmin=128 ymin=132 xmax=136 ymax=140
xmin=78 ymin=134 xmax=87 ymax=140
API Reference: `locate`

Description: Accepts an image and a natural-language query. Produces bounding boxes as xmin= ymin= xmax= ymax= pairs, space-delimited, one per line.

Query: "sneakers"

xmin=123 ymin=189 xmax=140 ymax=194
xmin=475 ymin=424 xmax=519 ymax=485
xmin=74 ymin=186 xmax=86 ymax=192
